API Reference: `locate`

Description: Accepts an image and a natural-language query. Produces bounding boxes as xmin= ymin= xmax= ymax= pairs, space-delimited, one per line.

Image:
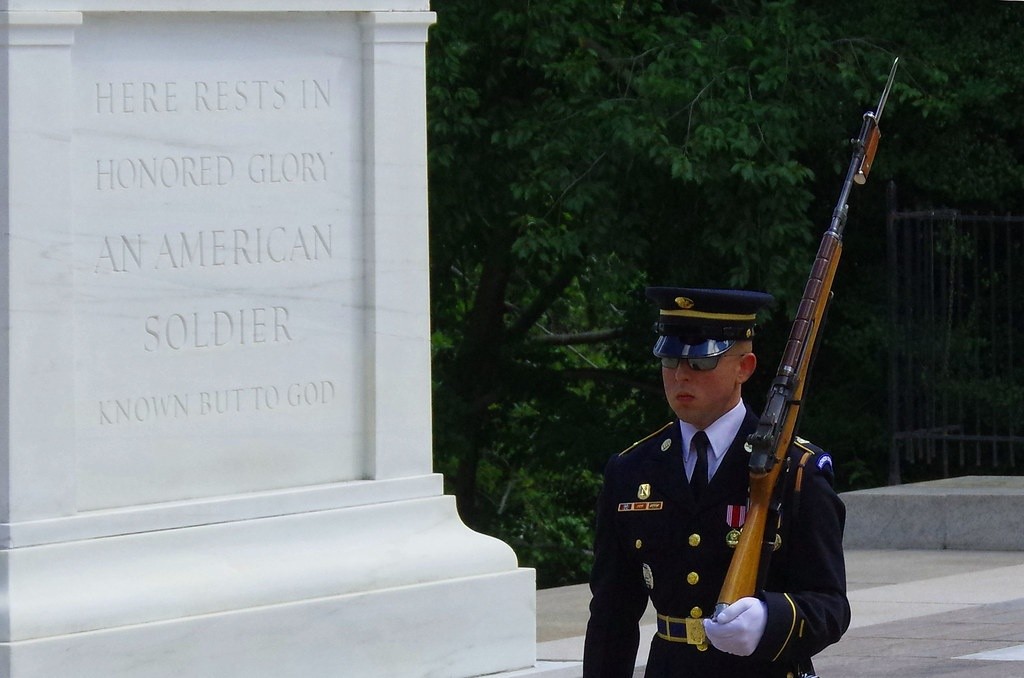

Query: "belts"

xmin=657 ymin=614 xmax=708 ymax=645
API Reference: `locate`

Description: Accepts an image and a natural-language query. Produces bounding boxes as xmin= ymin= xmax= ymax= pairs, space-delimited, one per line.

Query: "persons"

xmin=582 ymin=288 xmax=851 ymax=678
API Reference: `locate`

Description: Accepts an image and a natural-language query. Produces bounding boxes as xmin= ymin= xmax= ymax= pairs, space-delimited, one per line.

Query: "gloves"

xmin=703 ymin=597 xmax=768 ymax=656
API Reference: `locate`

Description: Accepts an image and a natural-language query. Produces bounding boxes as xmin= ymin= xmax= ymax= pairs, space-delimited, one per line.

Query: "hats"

xmin=644 ymin=285 xmax=774 ymax=358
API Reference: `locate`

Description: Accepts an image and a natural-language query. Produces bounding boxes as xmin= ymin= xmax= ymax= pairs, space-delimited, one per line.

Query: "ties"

xmin=689 ymin=432 xmax=711 ymax=503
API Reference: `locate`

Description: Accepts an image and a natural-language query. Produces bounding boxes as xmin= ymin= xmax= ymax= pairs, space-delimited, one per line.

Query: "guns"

xmin=711 ymin=56 xmax=900 ymax=626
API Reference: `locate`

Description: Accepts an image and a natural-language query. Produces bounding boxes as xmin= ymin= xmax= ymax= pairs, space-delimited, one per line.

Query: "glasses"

xmin=661 ymin=354 xmax=742 ymax=370
